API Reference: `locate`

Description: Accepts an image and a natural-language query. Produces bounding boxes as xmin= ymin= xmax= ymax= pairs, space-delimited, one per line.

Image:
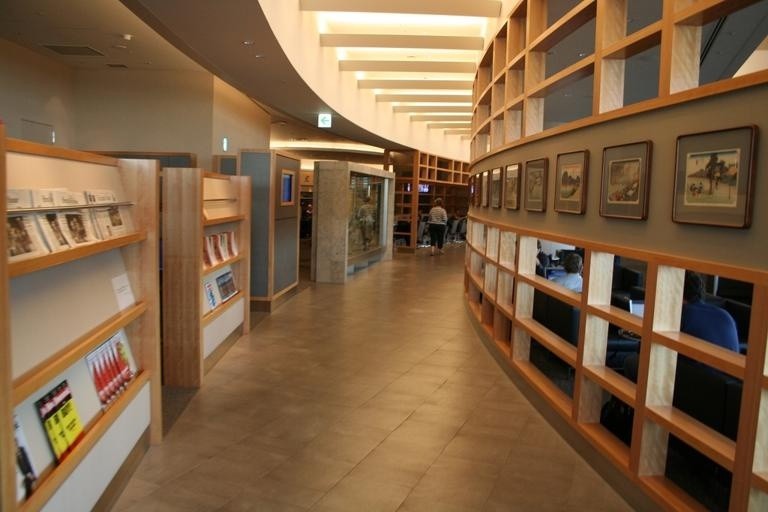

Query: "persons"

xmin=451 ymin=209 xmax=461 ymax=220
xmin=427 ymin=197 xmax=448 ymax=256
xmin=356 ymin=197 xmax=376 ymax=251
xmin=535 ymin=240 xmax=550 ymax=279
xmin=624 ymin=270 xmax=740 ymax=385
xmin=551 ymin=254 xmax=584 ymax=293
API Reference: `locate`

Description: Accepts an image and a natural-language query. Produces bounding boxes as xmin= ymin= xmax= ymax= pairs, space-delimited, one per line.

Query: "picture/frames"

xmin=670 ymin=124 xmax=758 ymax=229
xmin=553 ymin=149 xmax=589 ymax=215
xmin=467 ymin=163 xmax=521 ymax=210
xmin=523 ymin=157 xmax=547 ymax=213
xmin=597 ymin=140 xmax=651 ymax=221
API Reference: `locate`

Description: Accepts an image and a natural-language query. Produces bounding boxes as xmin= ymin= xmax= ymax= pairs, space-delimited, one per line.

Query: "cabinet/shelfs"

xmin=1 ymin=124 xmax=164 ymax=466
xmin=461 ymin=209 xmax=768 ymax=512
xmin=394 ymin=151 xmax=471 ymax=254
xmin=159 ymin=165 xmax=254 ymax=388
xmin=469 ymin=0 xmax=767 ymax=165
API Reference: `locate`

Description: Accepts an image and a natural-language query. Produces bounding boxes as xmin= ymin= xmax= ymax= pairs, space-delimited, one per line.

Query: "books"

xmin=202 ymin=231 xmax=239 ymax=311
xmin=12 ymin=332 xmax=136 ymax=506
xmin=7 ymin=187 xmax=126 ymax=263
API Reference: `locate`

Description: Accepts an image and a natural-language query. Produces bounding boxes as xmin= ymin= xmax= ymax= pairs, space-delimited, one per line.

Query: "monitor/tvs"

xmin=545 ymin=265 xmax=566 ymax=281
xmin=630 ymin=299 xmax=646 ymax=317
xmin=406 ymin=183 xmax=428 ymax=193
